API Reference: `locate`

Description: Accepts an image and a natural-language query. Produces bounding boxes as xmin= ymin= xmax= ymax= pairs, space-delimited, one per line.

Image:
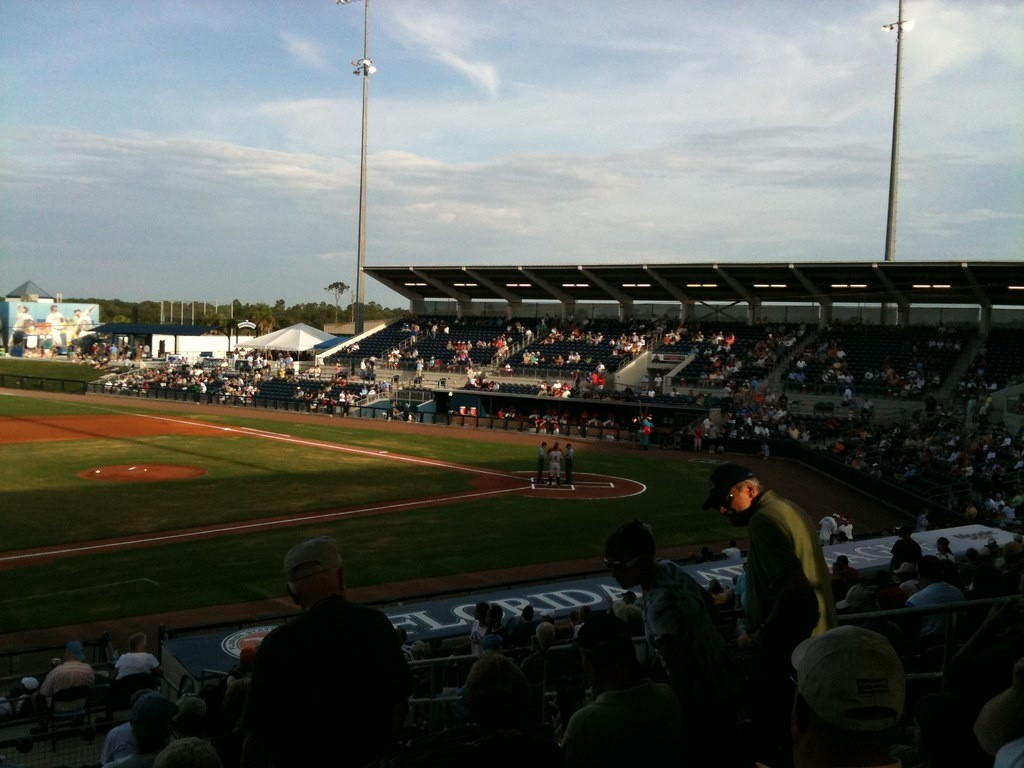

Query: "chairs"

xmin=38 ymin=314 xmax=1024 ymax=753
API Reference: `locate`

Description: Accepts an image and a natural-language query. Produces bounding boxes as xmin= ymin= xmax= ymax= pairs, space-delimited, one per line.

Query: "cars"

xmin=53 ymin=346 xmax=69 ymax=354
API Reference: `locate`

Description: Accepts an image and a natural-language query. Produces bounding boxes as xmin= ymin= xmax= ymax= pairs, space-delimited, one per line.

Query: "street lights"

xmin=883 ymin=18 xmax=919 ymax=262
xmin=351 ymin=60 xmax=377 ymax=340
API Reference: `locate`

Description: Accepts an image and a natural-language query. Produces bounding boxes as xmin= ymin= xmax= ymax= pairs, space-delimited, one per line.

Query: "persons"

xmin=0 ymin=461 xmax=1024 ymax=768
xmin=73 ymin=305 xmax=97 ymax=338
xmin=8 ymin=303 xmax=35 ymax=347
xmin=45 ymin=306 xmax=65 ymax=351
xmin=0 ymin=292 xmax=1024 ymax=492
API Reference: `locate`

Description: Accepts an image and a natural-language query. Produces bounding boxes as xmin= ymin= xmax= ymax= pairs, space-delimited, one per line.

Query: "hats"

xmin=131 ymin=692 xmax=177 ymax=727
xmin=175 ymin=693 xmax=206 ymax=717
xmin=66 ymin=640 xmax=85 ymax=660
xmin=702 ymin=463 xmax=753 ymax=511
xmin=791 ymin=625 xmax=906 ymax=731
xmin=622 ymin=591 xmax=637 ymax=600
xmin=835 ymin=584 xmax=869 ymax=609
xmin=284 ymin=536 xmax=342 ymax=582
xmin=21 ymin=677 xmax=38 ymax=690
xmin=892 ymin=562 xmax=914 ymax=574
xmin=575 ymin=612 xmax=632 ymax=649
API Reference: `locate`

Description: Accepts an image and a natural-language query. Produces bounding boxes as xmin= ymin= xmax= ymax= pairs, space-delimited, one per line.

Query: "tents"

xmin=237 ymin=323 xmax=338 ymax=359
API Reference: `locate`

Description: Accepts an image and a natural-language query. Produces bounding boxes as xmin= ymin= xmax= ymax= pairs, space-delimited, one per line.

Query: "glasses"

xmin=712 ymin=483 xmax=754 ymax=510
xmin=603 ymin=555 xmax=642 ymax=572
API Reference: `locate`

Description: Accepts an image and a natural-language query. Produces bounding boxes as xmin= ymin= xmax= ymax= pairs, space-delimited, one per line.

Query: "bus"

xmin=202 ymin=358 xmax=228 ymax=371
